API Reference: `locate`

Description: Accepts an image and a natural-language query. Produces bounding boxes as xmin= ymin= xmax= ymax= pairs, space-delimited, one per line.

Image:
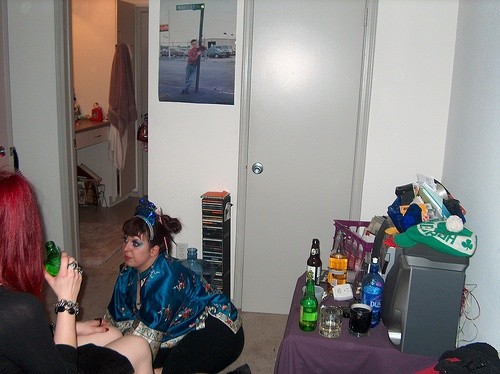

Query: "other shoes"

xmin=239 ymin=364 xmax=251 ymax=374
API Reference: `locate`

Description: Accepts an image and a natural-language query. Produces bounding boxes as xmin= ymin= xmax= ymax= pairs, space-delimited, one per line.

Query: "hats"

xmin=394 ymin=215 xmax=478 ymax=257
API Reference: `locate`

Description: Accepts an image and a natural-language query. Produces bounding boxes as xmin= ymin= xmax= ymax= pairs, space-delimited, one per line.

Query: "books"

xmin=202 ymin=192 xmax=230 ymax=290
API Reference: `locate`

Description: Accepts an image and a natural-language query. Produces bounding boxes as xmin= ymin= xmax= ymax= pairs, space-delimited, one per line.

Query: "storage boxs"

xmin=331 ymin=219 xmax=373 ymax=275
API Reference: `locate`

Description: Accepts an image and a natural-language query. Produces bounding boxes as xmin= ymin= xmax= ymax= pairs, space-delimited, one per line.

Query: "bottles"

xmin=298 ymin=279 xmax=319 ymax=331
xmin=180 ymin=247 xmax=216 ymax=289
xmin=73 ymin=97 xmax=81 ymax=126
xmin=92 ymin=102 xmax=104 ymax=123
xmin=306 ymin=239 xmax=323 ymax=285
xmin=43 ymin=241 xmax=60 ymax=276
xmin=358 ymin=262 xmax=385 ymax=328
xmin=326 ymin=232 xmax=349 ymax=295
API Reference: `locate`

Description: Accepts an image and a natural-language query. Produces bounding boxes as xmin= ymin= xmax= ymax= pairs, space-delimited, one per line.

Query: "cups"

xmin=348 ymin=303 xmax=373 ymax=339
xmin=319 ymin=305 xmax=344 ymax=339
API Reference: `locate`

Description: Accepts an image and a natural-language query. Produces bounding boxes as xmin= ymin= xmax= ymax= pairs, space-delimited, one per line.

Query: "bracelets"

xmin=55 ymin=299 xmax=79 ymax=315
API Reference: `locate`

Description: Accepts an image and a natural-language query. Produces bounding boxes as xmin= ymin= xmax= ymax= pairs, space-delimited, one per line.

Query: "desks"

xmin=275 ymin=271 xmax=439 ymax=374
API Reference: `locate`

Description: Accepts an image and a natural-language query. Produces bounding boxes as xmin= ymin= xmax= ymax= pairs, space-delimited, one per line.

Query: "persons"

xmin=0 ymin=171 xmax=154 ymax=374
xmin=103 ymin=199 xmax=252 ymax=374
xmin=181 ymin=39 xmax=206 ymax=94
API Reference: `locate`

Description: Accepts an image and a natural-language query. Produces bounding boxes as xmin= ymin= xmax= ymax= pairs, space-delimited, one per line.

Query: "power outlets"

xmin=175 ymin=242 xmax=188 ymax=260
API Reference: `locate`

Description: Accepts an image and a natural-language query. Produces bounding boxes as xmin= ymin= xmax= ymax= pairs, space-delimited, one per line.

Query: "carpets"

xmin=77 ymin=222 xmax=122 ymax=267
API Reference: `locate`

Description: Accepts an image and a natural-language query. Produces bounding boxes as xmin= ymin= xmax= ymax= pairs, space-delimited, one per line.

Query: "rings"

xmin=95 ymin=318 xmax=102 ymax=326
xmin=69 ymin=262 xmax=76 ymax=269
xmin=79 ymin=269 xmax=83 ymax=274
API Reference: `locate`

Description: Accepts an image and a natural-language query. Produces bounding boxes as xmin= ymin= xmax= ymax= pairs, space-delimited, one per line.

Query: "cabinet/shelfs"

xmin=74 ymin=118 xmax=109 ymax=150
xmin=116 ymin=0 xmax=137 ymax=197
